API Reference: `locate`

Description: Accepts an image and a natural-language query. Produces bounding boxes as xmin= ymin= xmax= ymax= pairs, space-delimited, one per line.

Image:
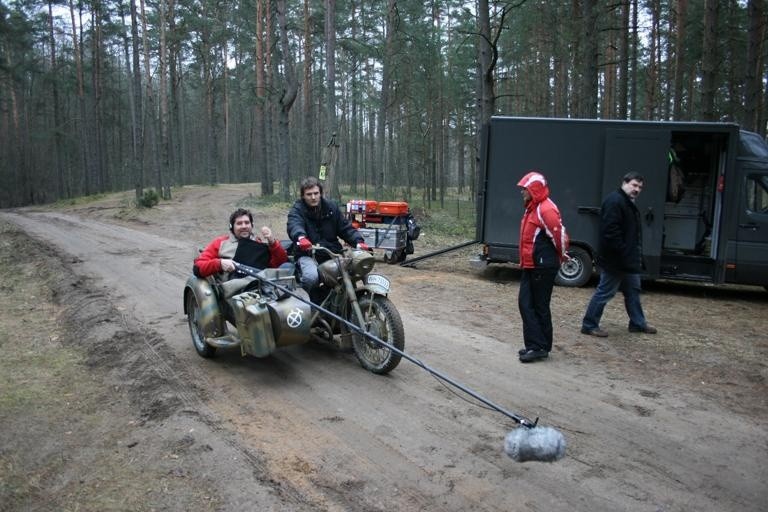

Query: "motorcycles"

xmin=182 ymin=234 xmax=408 ymax=376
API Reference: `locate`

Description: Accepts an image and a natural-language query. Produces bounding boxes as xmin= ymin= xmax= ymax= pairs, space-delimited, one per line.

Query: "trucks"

xmin=469 ymin=111 xmax=767 ymax=300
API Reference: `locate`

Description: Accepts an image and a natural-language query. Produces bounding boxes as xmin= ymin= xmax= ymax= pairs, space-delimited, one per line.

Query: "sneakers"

xmin=520 ymin=348 xmax=549 ymax=363
xmin=628 ymin=322 xmax=658 ymax=335
xmin=581 ymin=325 xmax=608 ymax=337
xmin=519 ymin=348 xmax=528 ymax=355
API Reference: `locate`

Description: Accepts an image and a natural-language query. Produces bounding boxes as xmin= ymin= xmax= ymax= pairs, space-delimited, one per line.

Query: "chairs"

xmin=278 ymin=238 xmax=297 ymax=263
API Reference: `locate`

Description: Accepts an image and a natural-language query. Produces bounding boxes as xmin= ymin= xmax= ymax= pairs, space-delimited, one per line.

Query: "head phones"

xmin=229 ymin=223 xmax=254 ymax=230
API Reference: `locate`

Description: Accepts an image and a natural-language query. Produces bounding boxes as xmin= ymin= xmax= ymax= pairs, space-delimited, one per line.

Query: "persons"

xmin=581 ymin=172 xmax=658 ymax=337
xmin=287 ymin=176 xmax=370 ymax=300
xmin=517 ymin=172 xmax=571 ymax=362
xmin=192 ymin=208 xmax=287 ymax=296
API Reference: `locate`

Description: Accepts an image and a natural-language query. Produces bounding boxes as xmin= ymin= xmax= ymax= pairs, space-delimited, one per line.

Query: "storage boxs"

xmin=348 ymin=198 xmax=409 ymax=250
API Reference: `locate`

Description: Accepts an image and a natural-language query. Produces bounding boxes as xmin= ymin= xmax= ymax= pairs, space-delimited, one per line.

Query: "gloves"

xmin=297 ymin=235 xmax=312 ymax=250
xmin=355 ymin=241 xmax=369 ymax=252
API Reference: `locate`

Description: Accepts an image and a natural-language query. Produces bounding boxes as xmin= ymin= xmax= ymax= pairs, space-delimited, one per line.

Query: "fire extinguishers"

xmin=718 ymin=174 xmax=724 ymax=193
